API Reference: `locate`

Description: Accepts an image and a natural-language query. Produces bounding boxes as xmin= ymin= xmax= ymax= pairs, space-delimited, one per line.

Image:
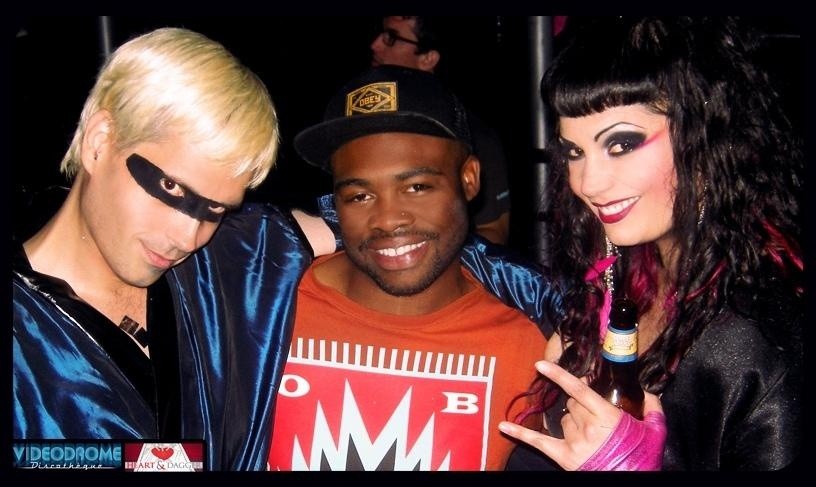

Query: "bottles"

xmin=588 ymin=298 xmax=645 ymax=425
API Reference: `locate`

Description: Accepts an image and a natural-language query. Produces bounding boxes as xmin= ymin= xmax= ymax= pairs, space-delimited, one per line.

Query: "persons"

xmin=493 ymin=2 xmax=816 ymax=469
xmin=244 ymin=61 xmax=571 ymax=471
xmin=364 ymin=3 xmax=514 ymax=254
xmin=6 ymin=22 xmax=564 ymax=472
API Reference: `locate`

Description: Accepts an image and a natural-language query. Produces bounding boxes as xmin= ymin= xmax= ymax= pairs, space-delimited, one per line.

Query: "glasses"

xmin=376 ymin=29 xmax=430 ymax=48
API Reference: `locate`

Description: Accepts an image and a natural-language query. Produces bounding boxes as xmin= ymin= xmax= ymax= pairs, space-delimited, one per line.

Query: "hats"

xmin=295 ymin=68 xmax=470 ymax=170
